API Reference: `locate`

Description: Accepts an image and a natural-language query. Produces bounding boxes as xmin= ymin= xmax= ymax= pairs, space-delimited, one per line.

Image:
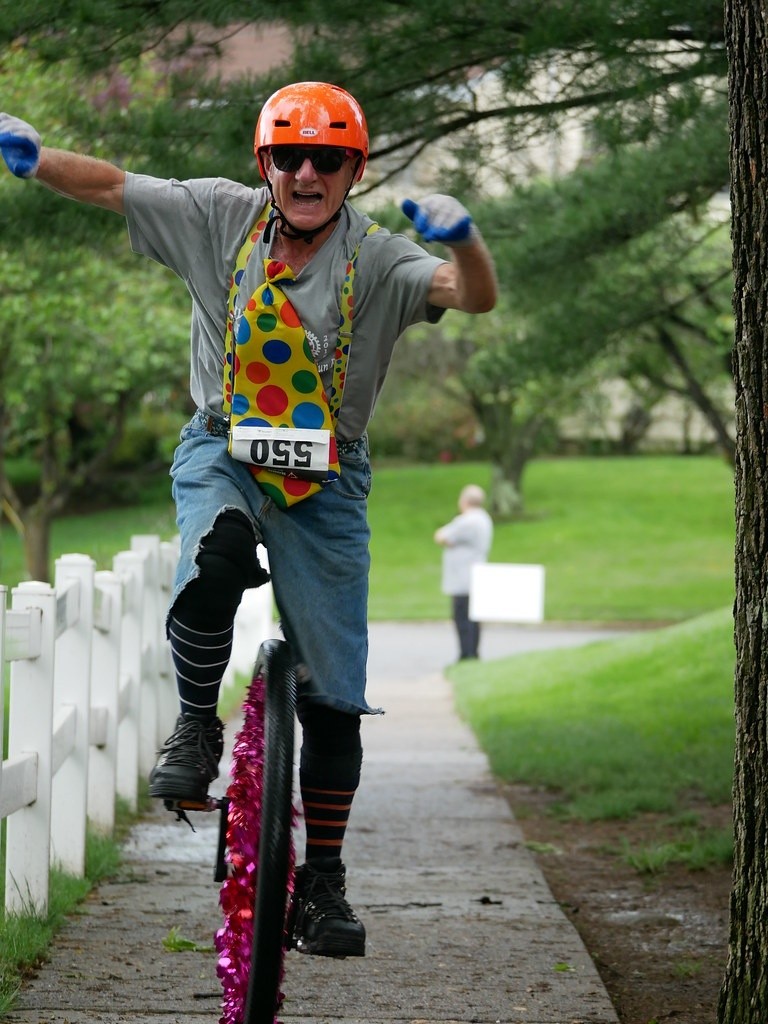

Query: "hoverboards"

xmin=163 ymin=637 xmax=350 ymax=1024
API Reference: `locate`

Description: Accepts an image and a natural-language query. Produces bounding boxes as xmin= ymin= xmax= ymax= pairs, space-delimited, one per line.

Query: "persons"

xmin=433 ymin=483 xmax=494 ymax=660
xmin=0 ymin=78 xmax=499 ymax=955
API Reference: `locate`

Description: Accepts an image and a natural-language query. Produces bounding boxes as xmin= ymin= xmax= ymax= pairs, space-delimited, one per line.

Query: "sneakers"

xmin=294 ymin=862 xmax=366 ymax=957
xmin=150 ymin=713 xmax=227 ymax=799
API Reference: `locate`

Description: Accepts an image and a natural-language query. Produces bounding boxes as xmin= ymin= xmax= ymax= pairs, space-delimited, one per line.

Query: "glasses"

xmin=264 ymin=145 xmax=360 ymax=175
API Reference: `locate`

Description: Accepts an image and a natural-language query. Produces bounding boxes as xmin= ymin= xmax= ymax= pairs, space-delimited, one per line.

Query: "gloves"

xmin=0 ymin=111 xmax=41 ymax=180
xmin=402 ymin=193 xmax=478 ymax=251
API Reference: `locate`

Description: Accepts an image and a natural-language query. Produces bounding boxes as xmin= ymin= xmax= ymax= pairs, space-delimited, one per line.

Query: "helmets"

xmin=255 ymin=82 xmax=368 ymax=182
